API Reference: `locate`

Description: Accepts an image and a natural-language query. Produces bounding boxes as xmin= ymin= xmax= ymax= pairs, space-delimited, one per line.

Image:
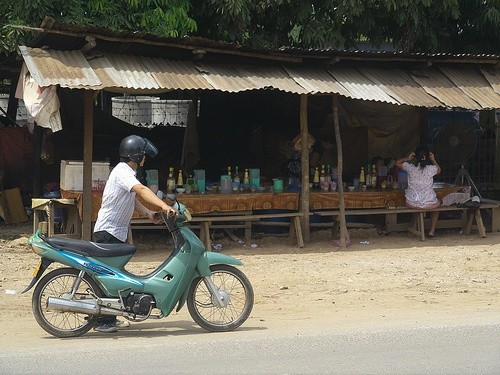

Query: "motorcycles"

xmin=21 ymin=190 xmax=254 ymax=338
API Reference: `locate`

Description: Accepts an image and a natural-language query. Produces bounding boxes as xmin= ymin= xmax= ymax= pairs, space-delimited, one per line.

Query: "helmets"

xmin=119 ymin=135 xmax=158 ymax=163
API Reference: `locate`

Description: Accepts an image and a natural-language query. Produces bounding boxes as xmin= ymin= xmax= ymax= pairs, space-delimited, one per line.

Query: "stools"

xmin=32 ymin=198 xmax=80 ymax=239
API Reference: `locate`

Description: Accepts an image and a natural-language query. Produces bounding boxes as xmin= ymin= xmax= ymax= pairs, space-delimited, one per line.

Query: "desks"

xmin=61 ymin=182 xmax=472 ymax=246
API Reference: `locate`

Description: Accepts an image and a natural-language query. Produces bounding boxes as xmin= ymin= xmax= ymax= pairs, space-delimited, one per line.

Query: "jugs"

xmin=146 ymin=170 xmax=158 ymax=189
xmin=221 ymin=175 xmax=232 ymax=194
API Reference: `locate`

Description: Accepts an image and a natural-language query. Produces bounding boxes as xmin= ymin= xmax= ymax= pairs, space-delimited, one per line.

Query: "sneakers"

xmin=113 ymin=320 xmax=131 ymax=327
xmin=93 ymin=323 xmax=117 ymax=332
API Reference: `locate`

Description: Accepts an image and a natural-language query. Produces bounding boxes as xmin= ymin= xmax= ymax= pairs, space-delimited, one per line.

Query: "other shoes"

xmin=429 ymin=231 xmax=435 ymax=236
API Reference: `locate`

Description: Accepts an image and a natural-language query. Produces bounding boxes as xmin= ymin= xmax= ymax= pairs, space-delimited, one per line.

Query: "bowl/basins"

xmin=349 ymin=187 xmax=354 ymax=191
xmin=176 ymin=188 xmax=186 ymax=193
xmin=257 ymin=187 xmax=266 ymax=192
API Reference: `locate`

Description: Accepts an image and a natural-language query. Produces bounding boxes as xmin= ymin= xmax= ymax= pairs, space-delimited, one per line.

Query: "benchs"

xmin=188 ymin=198 xmax=500 ymax=251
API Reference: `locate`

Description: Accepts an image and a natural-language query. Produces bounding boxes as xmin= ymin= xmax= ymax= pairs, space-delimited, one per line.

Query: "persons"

xmin=309 ymin=133 xmax=336 ymax=184
xmin=395 ymin=145 xmax=441 ymax=237
xmin=93 ymin=134 xmax=180 ymax=333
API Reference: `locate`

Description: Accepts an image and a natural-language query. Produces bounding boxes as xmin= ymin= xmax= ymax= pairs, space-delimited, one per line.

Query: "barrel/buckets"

xmin=43 ymin=191 xmax=70 ymax=228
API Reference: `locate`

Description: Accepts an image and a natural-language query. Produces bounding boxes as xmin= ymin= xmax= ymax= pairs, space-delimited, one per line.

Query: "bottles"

xmin=166 ymin=167 xmax=175 ymax=193
xmin=232 ymin=182 xmax=257 ymax=193
xmin=313 ymin=164 xmax=336 ymax=190
xmin=184 ymin=175 xmax=199 ymax=193
xmin=233 ymin=166 xmax=240 ymax=193
xmin=244 ymin=169 xmax=249 ymax=184
xmin=213 ymin=183 xmax=218 ymax=194
xmin=227 ymin=166 xmax=231 ymax=176
xmin=176 ymin=170 xmax=184 ymax=188
xmin=358 ymin=164 xmax=399 ymax=189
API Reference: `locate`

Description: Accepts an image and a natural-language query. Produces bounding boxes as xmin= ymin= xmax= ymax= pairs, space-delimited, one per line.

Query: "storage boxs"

xmin=60 ymin=160 xmax=111 ymax=193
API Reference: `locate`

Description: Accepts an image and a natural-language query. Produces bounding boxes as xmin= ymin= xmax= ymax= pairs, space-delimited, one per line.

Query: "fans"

xmin=439 ymin=126 xmax=483 ymax=198
